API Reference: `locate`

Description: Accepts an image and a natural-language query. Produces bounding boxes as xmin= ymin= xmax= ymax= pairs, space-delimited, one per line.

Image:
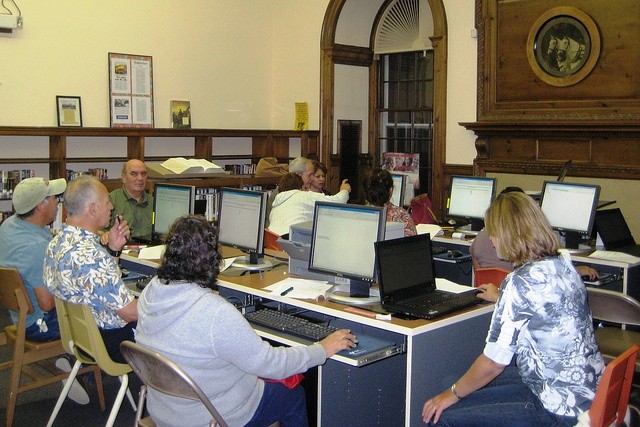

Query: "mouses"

xmin=581 ymin=274 xmax=598 ymax=282
xmin=120 ymin=267 xmax=129 ymax=281
xmin=348 ymin=338 xmax=360 ymax=347
xmin=473 ymin=290 xmax=488 ymax=303
xmin=449 ymin=249 xmax=463 ymax=259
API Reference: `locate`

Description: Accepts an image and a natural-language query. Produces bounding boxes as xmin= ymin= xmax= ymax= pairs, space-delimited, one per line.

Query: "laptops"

xmin=594 ymin=206 xmax=639 ymax=257
xmin=373 ymin=232 xmax=479 ymax=322
xmin=132 ymin=231 xmax=154 ymax=246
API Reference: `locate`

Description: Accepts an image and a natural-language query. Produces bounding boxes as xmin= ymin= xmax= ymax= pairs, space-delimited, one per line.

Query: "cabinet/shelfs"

xmin=1 ymin=126 xmax=319 ymax=224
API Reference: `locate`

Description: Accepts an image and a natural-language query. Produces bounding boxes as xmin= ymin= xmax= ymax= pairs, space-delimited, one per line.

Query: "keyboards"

xmin=135 ymin=271 xmax=157 ymax=290
xmin=245 ymin=307 xmax=339 ymax=342
xmin=431 ymin=242 xmax=447 ymax=255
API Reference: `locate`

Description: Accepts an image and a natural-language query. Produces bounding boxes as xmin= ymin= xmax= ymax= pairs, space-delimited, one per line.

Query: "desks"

xmin=431 ymin=226 xmax=639 ymax=331
xmin=116 ymin=236 xmax=498 ymax=427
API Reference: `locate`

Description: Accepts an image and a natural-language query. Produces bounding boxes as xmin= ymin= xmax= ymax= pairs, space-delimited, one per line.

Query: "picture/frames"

xmin=55 ymin=94 xmax=82 ymax=128
xmin=526 ymin=5 xmax=601 ymax=88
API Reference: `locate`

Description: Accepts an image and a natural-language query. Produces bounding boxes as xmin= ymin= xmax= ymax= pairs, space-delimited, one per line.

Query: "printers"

xmin=276 ymin=219 xmax=406 ymax=291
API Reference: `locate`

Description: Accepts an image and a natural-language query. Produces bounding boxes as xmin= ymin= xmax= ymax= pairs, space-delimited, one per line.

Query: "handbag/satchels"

xmin=409 ymin=195 xmax=440 ymax=224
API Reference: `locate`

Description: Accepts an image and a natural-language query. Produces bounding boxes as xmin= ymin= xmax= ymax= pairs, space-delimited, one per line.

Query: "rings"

xmin=432 ymin=407 xmax=436 ymax=410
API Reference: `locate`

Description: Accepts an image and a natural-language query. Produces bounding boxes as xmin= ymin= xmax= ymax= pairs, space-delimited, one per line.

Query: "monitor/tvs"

xmin=445 ymin=174 xmax=498 ymax=238
xmin=537 ymin=179 xmax=601 ymax=254
xmin=151 ymin=183 xmax=196 ymax=245
xmin=388 ymin=173 xmax=407 ymax=210
xmin=215 ymin=186 xmax=274 ymax=272
xmin=307 ymin=200 xmax=387 ymax=306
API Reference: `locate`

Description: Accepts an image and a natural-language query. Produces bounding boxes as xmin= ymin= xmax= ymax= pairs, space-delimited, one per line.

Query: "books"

xmin=1 ymin=170 xmax=35 ymax=200
xmin=160 ymin=157 xmax=225 ymax=175
xmin=170 ymin=100 xmax=191 ymax=128
xmin=225 ymin=164 xmax=256 ymax=174
xmin=66 ymin=166 xmax=107 ymax=180
xmin=243 ymin=185 xmax=262 ymax=191
xmin=196 ymin=187 xmax=220 ymax=223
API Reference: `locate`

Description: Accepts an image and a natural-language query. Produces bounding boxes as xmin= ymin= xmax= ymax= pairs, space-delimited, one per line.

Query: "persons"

xmin=393 ymin=157 xmax=404 ymax=171
xmin=398 ymin=156 xmax=416 ymax=171
xmin=421 ymin=190 xmax=607 ymax=426
xmin=469 ymin=186 xmax=600 ymax=295
xmin=134 ymin=215 xmax=358 ymax=426
xmin=97 ymin=159 xmax=155 ymax=247
xmin=363 ymin=169 xmax=417 ymax=238
xmin=268 ymin=172 xmax=352 ymax=242
xmin=43 ymin=176 xmax=158 ymax=426
xmin=0 ymin=177 xmax=90 ymax=405
xmin=311 ymin=161 xmax=332 ymax=196
xmin=265 ymin=157 xmax=314 ymax=227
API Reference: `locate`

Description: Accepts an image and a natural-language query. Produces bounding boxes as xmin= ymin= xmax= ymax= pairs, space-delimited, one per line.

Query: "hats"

xmin=12 ymin=177 xmax=67 ymax=214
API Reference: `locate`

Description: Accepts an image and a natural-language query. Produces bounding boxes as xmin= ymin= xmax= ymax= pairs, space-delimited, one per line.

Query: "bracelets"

xmin=451 ymin=384 xmax=463 ymax=400
xmin=107 ymin=243 xmax=122 ymax=257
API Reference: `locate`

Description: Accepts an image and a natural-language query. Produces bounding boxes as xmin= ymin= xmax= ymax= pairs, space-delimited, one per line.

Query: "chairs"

xmin=0 ymin=265 xmax=107 ymax=427
xmin=585 ymin=286 xmax=640 ymax=370
xmin=45 ymin=293 xmax=138 ymax=427
xmin=473 ymin=268 xmax=512 ymax=289
xmin=118 ymin=339 xmax=281 ymax=427
xmin=588 ymin=346 xmax=639 ymax=427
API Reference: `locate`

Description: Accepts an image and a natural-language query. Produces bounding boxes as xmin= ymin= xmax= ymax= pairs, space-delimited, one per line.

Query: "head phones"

xmin=222 ymin=293 xmax=243 ymax=307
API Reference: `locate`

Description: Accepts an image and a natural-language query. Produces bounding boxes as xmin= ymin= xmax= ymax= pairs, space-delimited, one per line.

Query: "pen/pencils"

xmin=116 ymin=215 xmax=129 ymax=242
xmin=281 ymin=287 xmax=293 ymax=296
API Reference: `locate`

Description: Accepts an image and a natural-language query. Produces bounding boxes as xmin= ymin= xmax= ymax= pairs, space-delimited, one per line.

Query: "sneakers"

xmin=53 ymin=353 xmax=91 ymax=405
